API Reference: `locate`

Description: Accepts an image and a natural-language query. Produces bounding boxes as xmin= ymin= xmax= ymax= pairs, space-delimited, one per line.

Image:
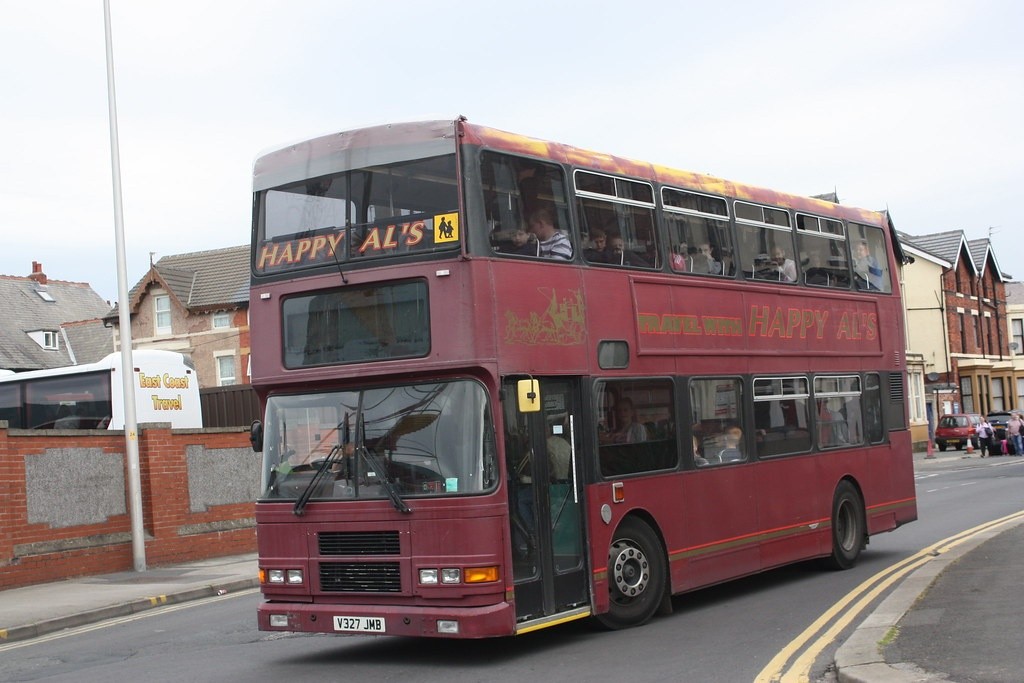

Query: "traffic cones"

xmin=924 ymin=439 xmax=936 ymax=459
xmin=964 ymin=432 xmax=976 ymax=453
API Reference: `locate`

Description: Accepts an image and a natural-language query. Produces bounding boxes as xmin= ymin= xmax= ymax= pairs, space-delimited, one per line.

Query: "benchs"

xmin=761 ymin=260 xmax=780 ymax=281
xmin=743 ymin=264 xmax=754 ymax=278
xmin=685 ymin=255 xmax=694 ymax=272
xmin=802 ymin=271 xmax=806 ymax=284
xmin=610 ymin=247 xmax=624 ymax=266
xmin=490 ymin=231 xmax=540 ymax=257
xmin=808 ymin=267 xmax=830 ymax=287
xmin=719 ymin=260 xmax=725 ymax=276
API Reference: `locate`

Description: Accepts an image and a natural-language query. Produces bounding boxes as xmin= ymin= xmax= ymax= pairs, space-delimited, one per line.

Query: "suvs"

xmin=935 ymin=413 xmax=987 ymax=451
xmin=985 ymin=409 xmax=1024 ymax=440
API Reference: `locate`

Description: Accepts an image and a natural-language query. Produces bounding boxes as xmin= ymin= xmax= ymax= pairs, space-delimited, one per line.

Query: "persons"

xmin=493 ymin=175 xmax=882 ymax=294
xmin=975 ymin=412 xmax=1024 ymax=458
xmin=517 ymin=378 xmax=878 ymax=549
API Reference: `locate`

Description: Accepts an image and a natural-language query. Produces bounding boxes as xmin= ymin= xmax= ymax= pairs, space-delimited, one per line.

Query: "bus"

xmin=247 ymin=117 xmax=919 ymax=638
xmin=0 ymin=350 xmax=204 ymax=431
